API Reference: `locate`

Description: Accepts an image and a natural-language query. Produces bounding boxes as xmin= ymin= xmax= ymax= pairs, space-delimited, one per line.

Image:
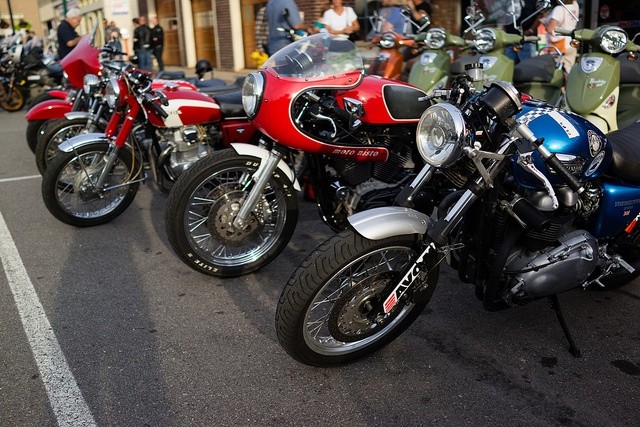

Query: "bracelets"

xmin=529 ymin=28 xmax=534 ymax=34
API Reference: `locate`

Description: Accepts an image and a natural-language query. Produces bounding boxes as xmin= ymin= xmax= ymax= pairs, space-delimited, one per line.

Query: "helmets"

xmin=195 ymin=60 xmax=213 ymax=76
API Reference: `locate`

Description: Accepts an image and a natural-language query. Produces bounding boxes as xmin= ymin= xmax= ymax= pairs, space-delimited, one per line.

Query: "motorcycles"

xmin=457 ymin=1 xmax=567 ymax=112
xmin=554 ymin=25 xmax=637 ymax=134
xmin=275 ymin=64 xmax=640 ymax=362
xmin=42 ymin=73 xmax=260 ymax=227
xmin=34 ymin=49 xmax=247 ymax=193
xmin=164 ymin=31 xmax=483 ymax=279
xmin=367 ymin=14 xmax=419 ymax=84
xmin=405 ymin=27 xmax=474 ymax=103
xmin=27 ymin=35 xmax=227 ymax=156
xmin=1 ymin=25 xmax=42 ymax=112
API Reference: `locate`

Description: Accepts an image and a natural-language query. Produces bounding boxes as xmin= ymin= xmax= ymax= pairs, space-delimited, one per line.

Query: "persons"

xmin=152 ymin=17 xmax=163 ymax=71
xmin=405 ymin=0 xmax=431 ymax=34
xmin=366 ymin=0 xmax=414 ymax=61
xmin=100 ymin=17 xmax=122 ymax=53
xmin=22 ymin=30 xmax=40 ymax=47
xmin=133 ymin=17 xmax=153 ymax=71
xmin=503 ymin=1 xmax=543 ymax=63
xmin=58 ymin=8 xmax=83 ymax=58
xmin=265 ymin=0 xmax=313 ymax=55
xmin=134 ymin=18 xmax=139 ymax=55
xmin=546 ymin=0 xmax=577 ymax=74
xmin=321 ymin=0 xmax=360 ymax=51
xmin=255 ymin=7 xmax=270 ymax=57
